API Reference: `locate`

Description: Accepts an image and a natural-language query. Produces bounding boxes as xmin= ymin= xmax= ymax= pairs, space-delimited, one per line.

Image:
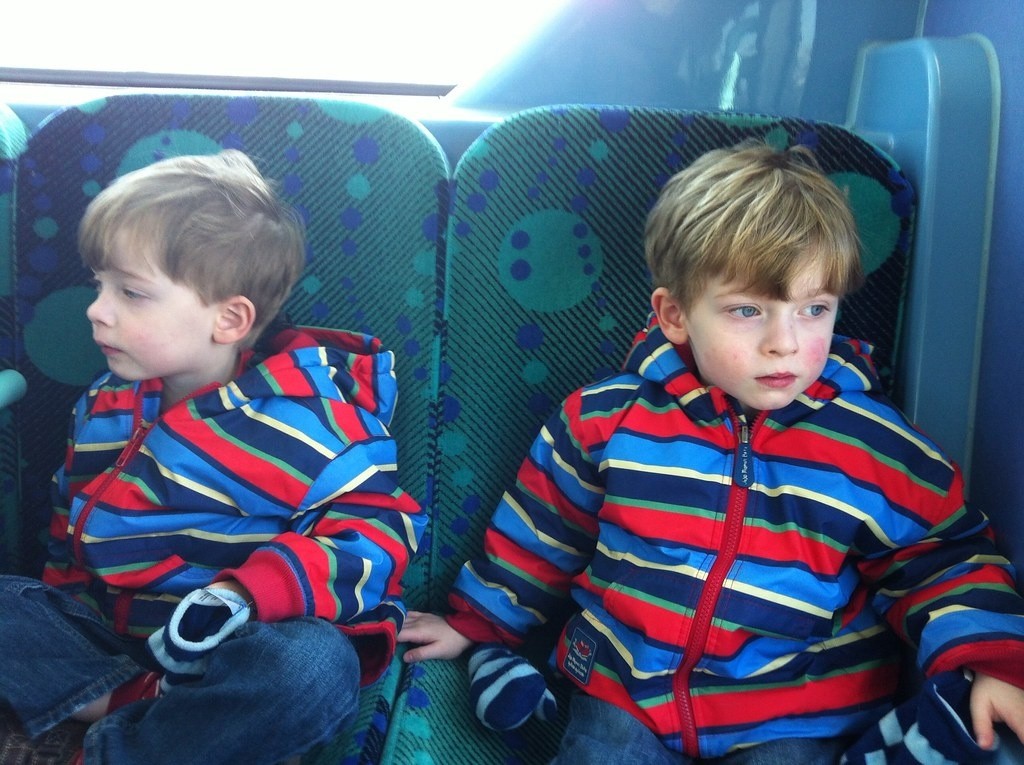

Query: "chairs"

xmin=0 ymin=91 xmax=1024 ymax=765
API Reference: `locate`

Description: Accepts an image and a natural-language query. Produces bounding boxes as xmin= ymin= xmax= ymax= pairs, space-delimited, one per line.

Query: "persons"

xmin=396 ymin=144 xmax=1024 ymax=764
xmin=0 ymin=148 xmax=430 ymax=765
